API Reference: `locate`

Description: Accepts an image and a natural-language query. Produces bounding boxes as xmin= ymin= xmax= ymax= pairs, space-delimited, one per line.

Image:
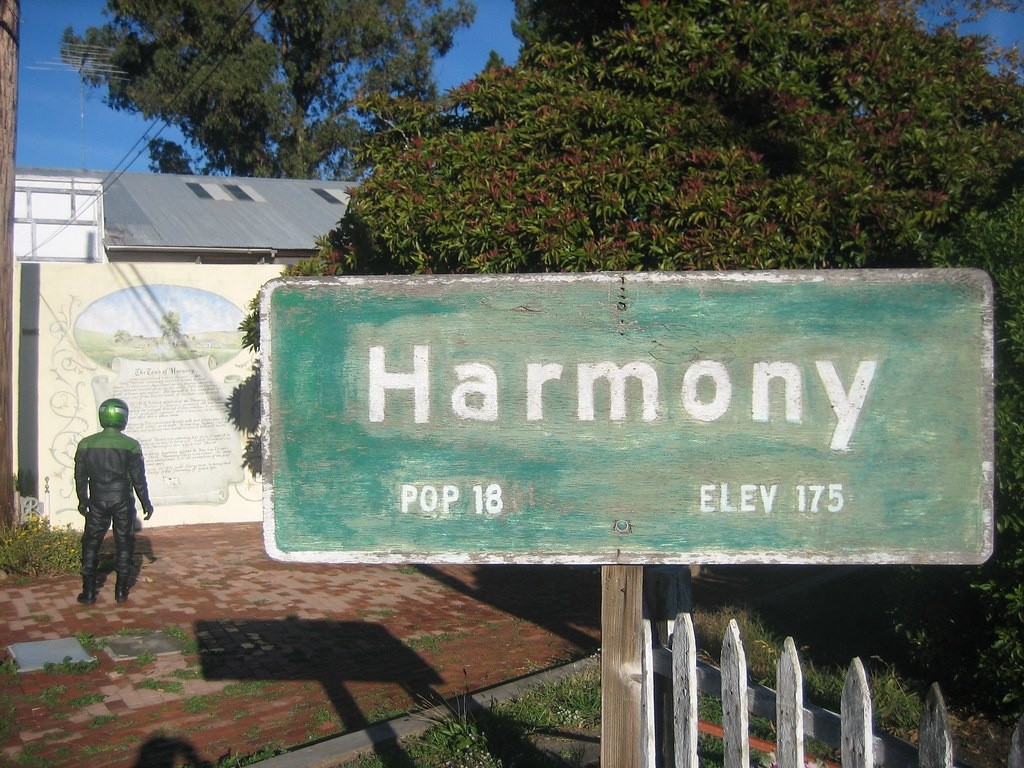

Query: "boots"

xmin=114 ymin=574 xmax=128 ymax=603
xmin=77 ymin=574 xmax=96 ymax=605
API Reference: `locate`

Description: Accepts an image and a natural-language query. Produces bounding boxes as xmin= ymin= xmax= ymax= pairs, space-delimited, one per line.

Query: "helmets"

xmin=98 ymin=398 xmax=129 ymax=430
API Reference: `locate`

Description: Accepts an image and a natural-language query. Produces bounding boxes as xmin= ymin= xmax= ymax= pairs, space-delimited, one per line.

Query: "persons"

xmin=72 ymin=398 xmax=153 ymax=605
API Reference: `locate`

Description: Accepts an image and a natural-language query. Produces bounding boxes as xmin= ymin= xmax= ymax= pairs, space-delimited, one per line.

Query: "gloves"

xmin=78 ymin=500 xmax=91 ymax=517
xmin=143 ymin=504 xmax=154 ymax=520
